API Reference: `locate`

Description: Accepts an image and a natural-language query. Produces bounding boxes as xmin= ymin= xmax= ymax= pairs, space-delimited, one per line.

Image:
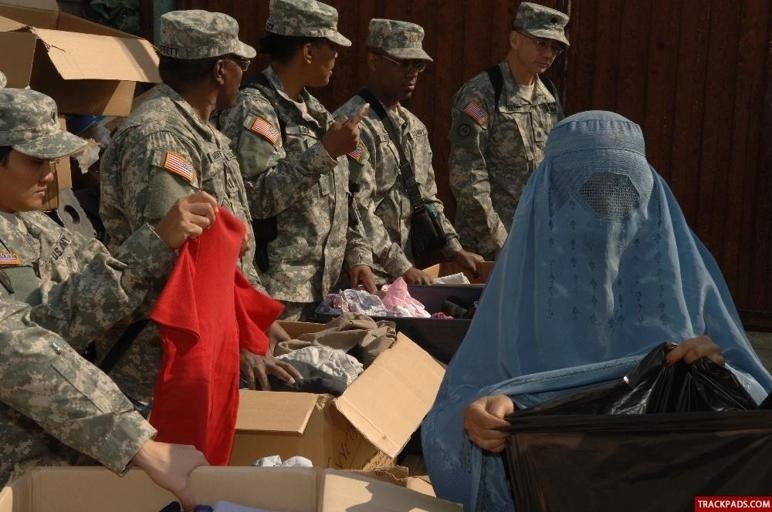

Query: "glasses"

xmin=517 ymin=32 xmax=565 ymax=53
xmin=382 ymin=55 xmax=426 ymax=73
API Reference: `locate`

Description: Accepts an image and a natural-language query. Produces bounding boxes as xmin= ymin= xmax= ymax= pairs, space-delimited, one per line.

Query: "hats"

xmin=513 ymin=2 xmax=570 ymax=47
xmin=160 ymin=0 xmax=434 ymax=62
xmin=0 ymin=88 xmax=89 ymax=159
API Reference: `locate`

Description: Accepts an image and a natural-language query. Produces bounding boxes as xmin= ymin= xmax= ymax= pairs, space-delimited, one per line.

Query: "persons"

xmin=223 ymin=0 xmax=378 ymax=321
xmin=332 ymin=18 xmax=485 ymax=290
xmin=1 ymin=290 xmax=212 ymax=510
xmin=448 ymin=2 xmax=573 ymax=263
xmin=0 ymin=88 xmax=220 ymax=477
xmin=98 ymin=11 xmax=304 ymax=393
xmin=420 ymin=111 xmax=771 ymax=512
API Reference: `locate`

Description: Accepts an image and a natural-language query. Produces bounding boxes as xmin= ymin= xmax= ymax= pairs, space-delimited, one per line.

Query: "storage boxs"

xmin=0 ymin=0 xmax=166 ymax=213
xmin=0 ymin=255 xmax=494 ymax=512
